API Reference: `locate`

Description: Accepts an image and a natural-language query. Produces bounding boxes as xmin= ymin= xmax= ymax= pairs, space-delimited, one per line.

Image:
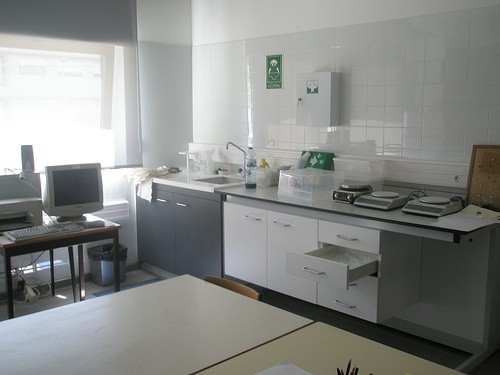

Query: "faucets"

xmin=226 ymin=142 xmax=246 ymax=172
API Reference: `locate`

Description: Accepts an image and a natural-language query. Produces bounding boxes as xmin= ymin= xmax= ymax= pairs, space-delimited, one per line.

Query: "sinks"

xmin=194 ymin=175 xmax=244 ymax=185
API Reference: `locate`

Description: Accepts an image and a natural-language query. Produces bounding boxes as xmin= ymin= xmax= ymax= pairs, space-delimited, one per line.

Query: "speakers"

xmin=21 ymin=145 xmax=35 ymax=173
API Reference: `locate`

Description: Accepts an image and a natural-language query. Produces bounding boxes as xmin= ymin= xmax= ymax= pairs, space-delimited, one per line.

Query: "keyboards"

xmin=3 ymin=222 xmax=82 ymax=242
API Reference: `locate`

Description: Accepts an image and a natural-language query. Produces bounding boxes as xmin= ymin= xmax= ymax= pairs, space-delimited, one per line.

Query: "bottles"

xmin=256 ymin=157 xmax=272 ymax=187
xmin=244 ymin=146 xmax=256 ymax=189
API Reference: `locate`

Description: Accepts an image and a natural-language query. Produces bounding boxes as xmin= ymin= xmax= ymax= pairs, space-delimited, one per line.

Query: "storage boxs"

xmin=333 ymin=157 xmax=386 ymax=191
xmin=277 ymin=168 xmax=345 ymax=201
xmin=250 ymin=163 xmax=290 ymax=186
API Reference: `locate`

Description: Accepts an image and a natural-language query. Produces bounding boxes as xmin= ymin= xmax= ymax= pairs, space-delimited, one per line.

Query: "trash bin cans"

xmin=87 ymin=243 xmax=128 ymax=287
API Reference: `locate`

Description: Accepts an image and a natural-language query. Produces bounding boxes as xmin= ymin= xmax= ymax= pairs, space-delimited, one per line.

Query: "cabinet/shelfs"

xmin=134 ymin=182 xmax=221 ymax=277
xmin=222 ymin=200 xmax=500 ymax=356
xmin=296 ymin=72 xmax=341 ymax=127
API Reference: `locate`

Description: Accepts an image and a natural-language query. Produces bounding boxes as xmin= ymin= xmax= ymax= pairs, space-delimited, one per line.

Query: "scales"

xmin=402 ymin=197 xmax=463 ymax=218
xmin=354 ymin=191 xmax=408 ymax=211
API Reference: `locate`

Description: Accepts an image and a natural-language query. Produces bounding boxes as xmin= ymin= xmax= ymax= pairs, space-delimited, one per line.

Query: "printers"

xmin=0 ymin=173 xmax=43 ymax=234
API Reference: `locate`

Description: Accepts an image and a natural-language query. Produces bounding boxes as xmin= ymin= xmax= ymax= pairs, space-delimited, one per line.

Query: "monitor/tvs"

xmin=45 ymin=163 xmax=104 ymax=221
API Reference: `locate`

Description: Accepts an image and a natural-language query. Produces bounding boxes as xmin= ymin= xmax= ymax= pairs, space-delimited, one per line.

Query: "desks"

xmin=0 ymin=274 xmax=313 ymax=375
xmin=0 ymin=213 xmax=122 ymax=319
xmin=193 ymin=322 xmax=469 ymax=375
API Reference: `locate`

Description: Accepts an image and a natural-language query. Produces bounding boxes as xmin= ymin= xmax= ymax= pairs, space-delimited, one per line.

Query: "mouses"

xmin=93 ymin=221 xmax=105 ymax=227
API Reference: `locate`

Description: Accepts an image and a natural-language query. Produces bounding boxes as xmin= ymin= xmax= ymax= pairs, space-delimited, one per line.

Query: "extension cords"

xmin=22 ymin=285 xmax=36 ymax=301
